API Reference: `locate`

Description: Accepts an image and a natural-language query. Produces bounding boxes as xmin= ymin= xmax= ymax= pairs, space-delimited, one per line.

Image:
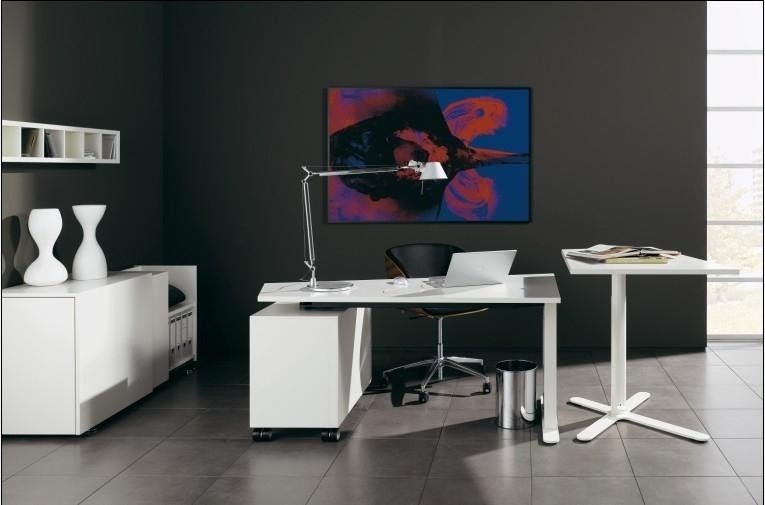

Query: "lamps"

xmin=299 ymin=161 xmax=448 ymax=287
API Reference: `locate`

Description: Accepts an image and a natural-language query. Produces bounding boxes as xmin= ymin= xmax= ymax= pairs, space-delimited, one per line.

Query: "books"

xmin=564 ymin=240 xmax=680 ymax=261
xmin=103 ymin=139 xmax=116 ymax=159
xmin=604 ymin=256 xmax=668 ymax=265
xmin=21 ymin=128 xmax=58 ymax=158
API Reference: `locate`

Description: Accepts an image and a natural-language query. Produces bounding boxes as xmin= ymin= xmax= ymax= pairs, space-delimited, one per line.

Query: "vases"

xmin=71 ymin=205 xmax=107 ymax=279
xmin=23 ymin=206 xmax=69 ymax=287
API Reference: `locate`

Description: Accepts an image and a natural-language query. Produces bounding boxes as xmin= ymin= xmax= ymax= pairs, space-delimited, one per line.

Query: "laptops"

xmin=423 ymin=250 xmax=517 ymax=287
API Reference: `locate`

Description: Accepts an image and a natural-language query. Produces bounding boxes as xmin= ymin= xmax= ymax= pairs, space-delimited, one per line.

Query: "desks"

xmin=247 ymin=271 xmax=562 ymax=445
xmin=560 ymin=246 xmax=707 ymax=442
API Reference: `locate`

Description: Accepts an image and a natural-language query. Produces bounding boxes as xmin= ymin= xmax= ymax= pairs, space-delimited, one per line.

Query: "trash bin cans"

xmin=495 ymin=359 xmax=539 ymax=430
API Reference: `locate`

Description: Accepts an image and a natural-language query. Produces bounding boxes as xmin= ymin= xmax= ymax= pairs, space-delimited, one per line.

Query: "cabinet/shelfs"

xmin=2 ymin=119 xmax=121 ymax=164
xmin=3 ymin=263 xmax=198 ymax=437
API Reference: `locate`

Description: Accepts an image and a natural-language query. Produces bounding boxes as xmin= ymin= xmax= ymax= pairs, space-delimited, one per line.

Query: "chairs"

xmin=377 ymin=243 xmax=490 ymax=404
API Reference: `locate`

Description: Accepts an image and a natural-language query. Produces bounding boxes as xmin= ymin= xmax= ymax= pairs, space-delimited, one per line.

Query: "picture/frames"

xmin=321 ymin=83 xmax=532 ymax=225
